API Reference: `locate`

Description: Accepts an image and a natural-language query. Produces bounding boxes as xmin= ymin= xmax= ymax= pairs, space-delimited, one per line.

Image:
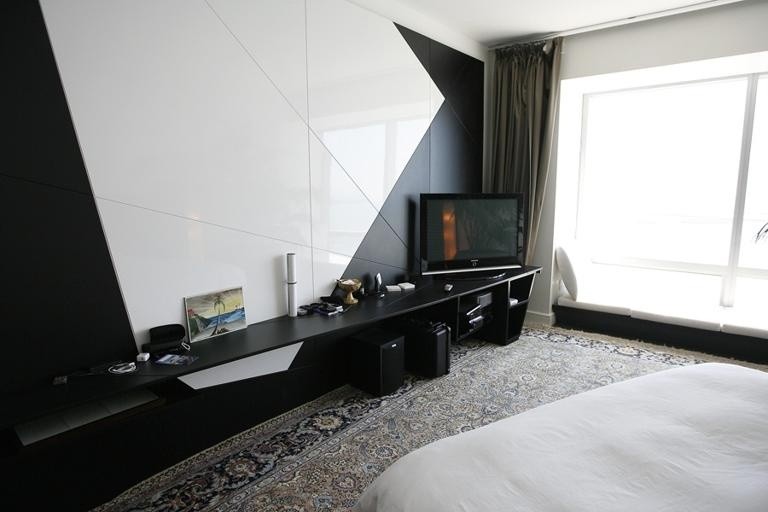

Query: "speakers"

xmin=341 ymin=328 xmax=406 ymax=398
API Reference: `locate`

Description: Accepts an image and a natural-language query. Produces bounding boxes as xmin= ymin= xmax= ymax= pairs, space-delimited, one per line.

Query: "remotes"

xmin=444 ymin=284 xmax=453 ymax=291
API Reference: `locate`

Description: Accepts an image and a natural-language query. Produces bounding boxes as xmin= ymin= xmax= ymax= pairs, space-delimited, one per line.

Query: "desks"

xmin=0 ymin=265 xmax=542 ymax=432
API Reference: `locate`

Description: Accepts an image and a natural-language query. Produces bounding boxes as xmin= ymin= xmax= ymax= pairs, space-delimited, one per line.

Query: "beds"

xmin=354 ymin=362 xmax=767 ymax=510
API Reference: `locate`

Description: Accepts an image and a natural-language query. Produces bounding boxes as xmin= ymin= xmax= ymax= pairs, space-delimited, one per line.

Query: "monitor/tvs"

xmin=419 ymin=191 xmax=529 ymax=281
